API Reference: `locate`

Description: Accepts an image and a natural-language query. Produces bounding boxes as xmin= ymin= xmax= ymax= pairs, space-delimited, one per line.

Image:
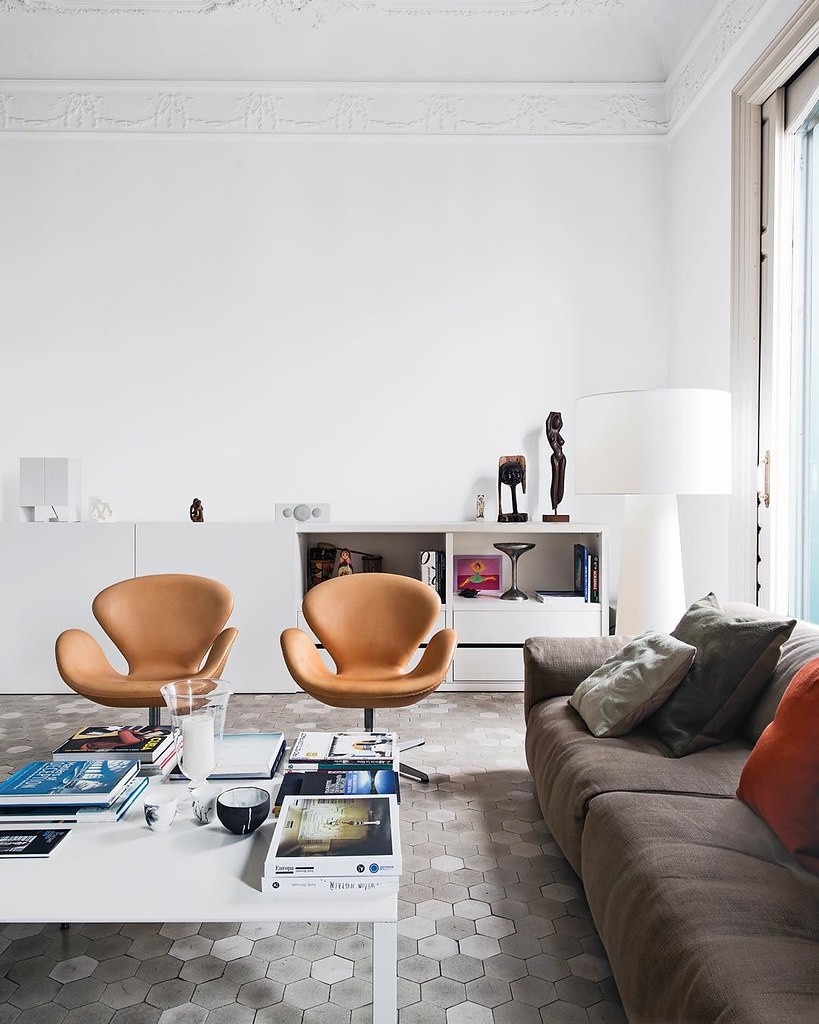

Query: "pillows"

xmin=637 ymin=591 xmax=797 ymax=758
xmin=569 ymin=625 xmax=697 ymax=739
xmin=736 ymin=658 xmax=819 ymax=875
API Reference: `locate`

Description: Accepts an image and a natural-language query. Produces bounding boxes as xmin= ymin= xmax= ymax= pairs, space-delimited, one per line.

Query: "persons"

xmin=0 ymin=841 xmax=29 ymax=847
xmin=338 ymin=548 xmax=354 ymax=576
xmin=546 ymin=411 xmax=566 ymax=509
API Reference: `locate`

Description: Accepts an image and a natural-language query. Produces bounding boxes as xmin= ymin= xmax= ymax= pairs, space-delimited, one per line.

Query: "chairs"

xmin=280 ymin=573 xmax=457 ymax=784
xmin=55 ymin=573 xmax=238 ymax=725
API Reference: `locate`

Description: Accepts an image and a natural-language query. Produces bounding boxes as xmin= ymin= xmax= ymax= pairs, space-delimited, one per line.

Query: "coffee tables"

xmin=0 ymin=747 xmax=403 ymax=1024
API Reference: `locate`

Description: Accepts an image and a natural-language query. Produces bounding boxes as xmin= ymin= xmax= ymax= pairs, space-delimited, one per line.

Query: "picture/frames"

xmin=453 ymin=555 xmax=504 ymax=596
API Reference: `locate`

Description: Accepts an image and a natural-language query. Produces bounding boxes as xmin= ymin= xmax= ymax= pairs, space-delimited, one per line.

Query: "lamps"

xmin=573 ymin=387 xmax=732 ymax=635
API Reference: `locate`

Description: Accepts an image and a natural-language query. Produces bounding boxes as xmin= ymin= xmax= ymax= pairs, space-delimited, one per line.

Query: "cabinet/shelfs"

xmin=0 ymin=520 xmax=607 ymax=694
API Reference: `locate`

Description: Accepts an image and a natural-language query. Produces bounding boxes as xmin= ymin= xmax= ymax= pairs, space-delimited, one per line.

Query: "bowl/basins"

xmin=216 ymin=787 xmax=270 ymax=834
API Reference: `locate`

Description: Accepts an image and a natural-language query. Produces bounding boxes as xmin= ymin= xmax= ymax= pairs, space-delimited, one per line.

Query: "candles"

xmin=160 ymin=678 xmax=233 ymax=814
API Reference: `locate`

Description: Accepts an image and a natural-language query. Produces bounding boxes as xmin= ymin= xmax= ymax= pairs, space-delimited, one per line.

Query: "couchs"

xmin=523 ymin=600 xmax=819 ymax=1024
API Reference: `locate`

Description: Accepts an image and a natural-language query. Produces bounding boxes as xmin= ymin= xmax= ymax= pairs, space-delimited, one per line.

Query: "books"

xmin=170 ymin=732 xmax=287 ymax=781
xmin=533 ymin=591 xmax=585 ymax=604
xmin=0 ymin=829 xmax=73 ymax=859
xmin=415 ymin=550 xmax=446 ymax=592
xmin=0 ymin=760 xmax=149 ymax=823
xmin=573 ymin=543 xmax=599 ymax=603
xmin=260 ymin=731 xmax=403 ymax=892
xmin=52 ymin=724 xmax=184 ymax=776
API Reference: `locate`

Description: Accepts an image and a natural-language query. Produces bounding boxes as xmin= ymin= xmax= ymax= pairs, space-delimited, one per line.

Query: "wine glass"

xmin=161 ymin=680 xmax=232 ymax=813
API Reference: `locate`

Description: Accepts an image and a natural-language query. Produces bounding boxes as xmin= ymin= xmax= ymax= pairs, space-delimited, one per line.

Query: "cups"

xmin=191 ymin=787 xmax=221 ymax=825
xmin=144 ymin=796 xmax=178 ymax=831
